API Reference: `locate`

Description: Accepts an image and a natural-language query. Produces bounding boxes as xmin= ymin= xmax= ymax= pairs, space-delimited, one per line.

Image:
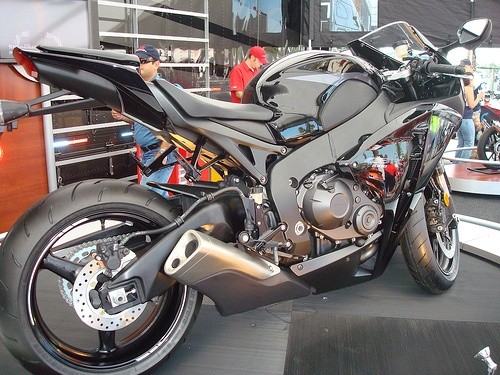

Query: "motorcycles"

xmin=477 ymin=102 xmax=500 ymax=170
xmin=1 ymin=18 xmax=494 ymax=375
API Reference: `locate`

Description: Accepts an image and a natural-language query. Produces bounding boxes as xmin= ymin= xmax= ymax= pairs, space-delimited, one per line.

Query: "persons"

xmin=111 ymin=44 xmax=183 ymax=198
xmin=460 ymin=59 xmax=481 ymax=146
xmin=453 ymin=72 xmax=484 ymax=164
xmin=229 ymin=46 xmax=269 ymax=103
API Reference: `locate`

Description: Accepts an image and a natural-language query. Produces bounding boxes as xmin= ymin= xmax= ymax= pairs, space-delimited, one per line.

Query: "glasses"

xmin=139 ymin=60 xmax=156 ymax=64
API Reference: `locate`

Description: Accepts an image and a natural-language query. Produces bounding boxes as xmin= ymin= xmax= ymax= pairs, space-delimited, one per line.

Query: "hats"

xmin=134 ymin=44 xmax=160 ymax=59
xmin=248 ymin=46 xmax=268 ymax=65
xmin=461 ymin=59 xmax=471 ymax=64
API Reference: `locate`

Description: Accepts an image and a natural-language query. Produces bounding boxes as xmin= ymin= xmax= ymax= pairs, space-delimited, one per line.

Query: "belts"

xmin=140 ymin=140 xmax=161 ymax=151
